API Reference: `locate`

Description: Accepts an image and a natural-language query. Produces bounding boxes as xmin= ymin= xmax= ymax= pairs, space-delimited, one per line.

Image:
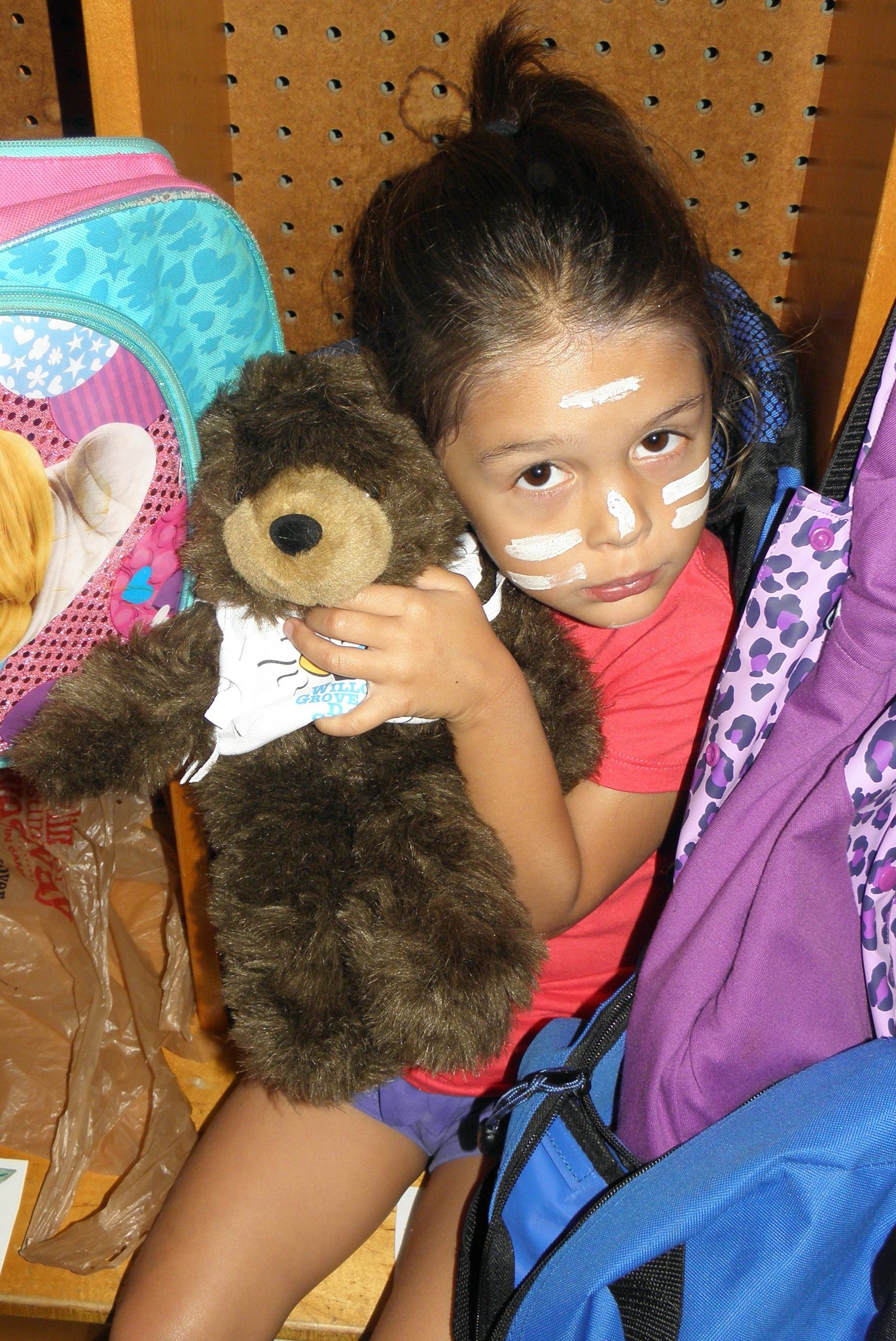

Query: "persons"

xmin=100 ymin=5 xmax=760 ymax=1341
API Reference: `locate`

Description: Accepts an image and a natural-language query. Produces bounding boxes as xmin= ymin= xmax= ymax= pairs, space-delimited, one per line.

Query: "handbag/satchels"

xmin=617 ymin=301 xmax=896 ymax=1160
xmin=460 ymin=988 xmax=896 ymax=1341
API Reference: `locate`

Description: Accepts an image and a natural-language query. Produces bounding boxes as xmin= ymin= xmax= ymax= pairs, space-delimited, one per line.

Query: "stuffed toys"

xmin=12 ymin=343 xmax=607 ymax=1117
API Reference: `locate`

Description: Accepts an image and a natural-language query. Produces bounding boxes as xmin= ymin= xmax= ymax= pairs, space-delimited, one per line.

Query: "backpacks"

xmin=0 ymin=136 xmax=287 ymax=758
